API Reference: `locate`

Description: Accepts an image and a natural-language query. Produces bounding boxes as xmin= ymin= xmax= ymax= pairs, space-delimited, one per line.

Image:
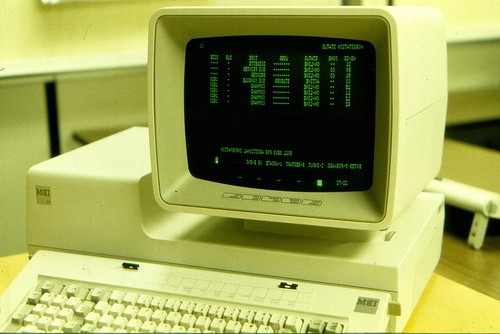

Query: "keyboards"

xmin=0 ymin=250 xmax=391 ymax=334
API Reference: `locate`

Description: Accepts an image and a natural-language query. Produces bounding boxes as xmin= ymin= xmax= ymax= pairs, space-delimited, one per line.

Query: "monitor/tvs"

xmin=147 ymin=6 xmax=448 ymax=241
